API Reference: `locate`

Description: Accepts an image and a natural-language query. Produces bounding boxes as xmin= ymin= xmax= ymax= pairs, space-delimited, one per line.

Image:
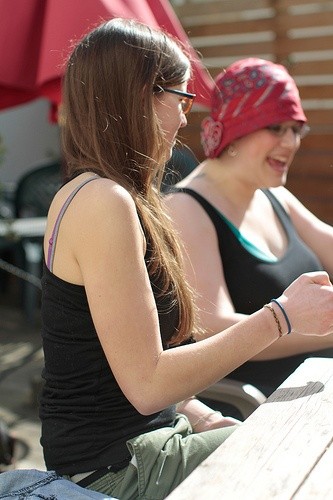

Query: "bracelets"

xmin=264 ymin=304 xmax=282 ymax=339
xmin=271 ymin=298 xmax=291 ymax=335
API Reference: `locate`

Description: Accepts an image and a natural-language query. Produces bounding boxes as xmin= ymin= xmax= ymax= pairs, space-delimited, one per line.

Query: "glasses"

xmin=146 ymin=79 xmax=195 ymax=115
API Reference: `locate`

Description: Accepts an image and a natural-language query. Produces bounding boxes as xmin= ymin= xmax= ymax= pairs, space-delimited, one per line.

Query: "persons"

xmin=158 ymin=56 xmax=333 ymax=421
xmin=41 ymin=16 xmax=333 ymax=500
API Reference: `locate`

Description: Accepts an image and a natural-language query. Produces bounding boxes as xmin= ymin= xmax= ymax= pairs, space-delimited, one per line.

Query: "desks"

xmin=160 ymin=357 xmax=333 ymax=500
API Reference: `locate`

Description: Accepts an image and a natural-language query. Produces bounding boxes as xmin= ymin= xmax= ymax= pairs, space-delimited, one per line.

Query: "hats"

xmin=200 ymin=56 xmax=307 ymax=159
